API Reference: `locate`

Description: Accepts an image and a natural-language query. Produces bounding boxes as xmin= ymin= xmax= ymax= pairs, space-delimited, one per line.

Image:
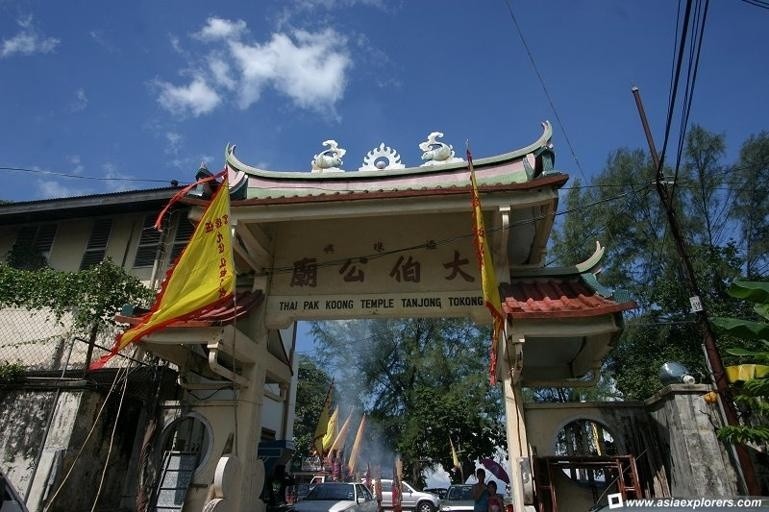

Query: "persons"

xmin=473 ymin=468 xmax=490 ymax=511
xmin=486 ymin=480 xmax=504 ymax=512
xmin=505 ymin=485 xmax=513 ymax=512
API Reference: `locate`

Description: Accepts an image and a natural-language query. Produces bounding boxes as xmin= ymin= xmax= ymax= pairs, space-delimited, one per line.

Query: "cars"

xmin=285 ymin=472 xmax=474 ymax=512
xmin=587 ymin=495 xmax=769 ymax=512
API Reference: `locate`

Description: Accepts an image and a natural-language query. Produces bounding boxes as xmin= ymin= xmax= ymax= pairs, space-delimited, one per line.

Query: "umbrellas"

xmin=482 ymin=458 xmax=509 ymax=484
